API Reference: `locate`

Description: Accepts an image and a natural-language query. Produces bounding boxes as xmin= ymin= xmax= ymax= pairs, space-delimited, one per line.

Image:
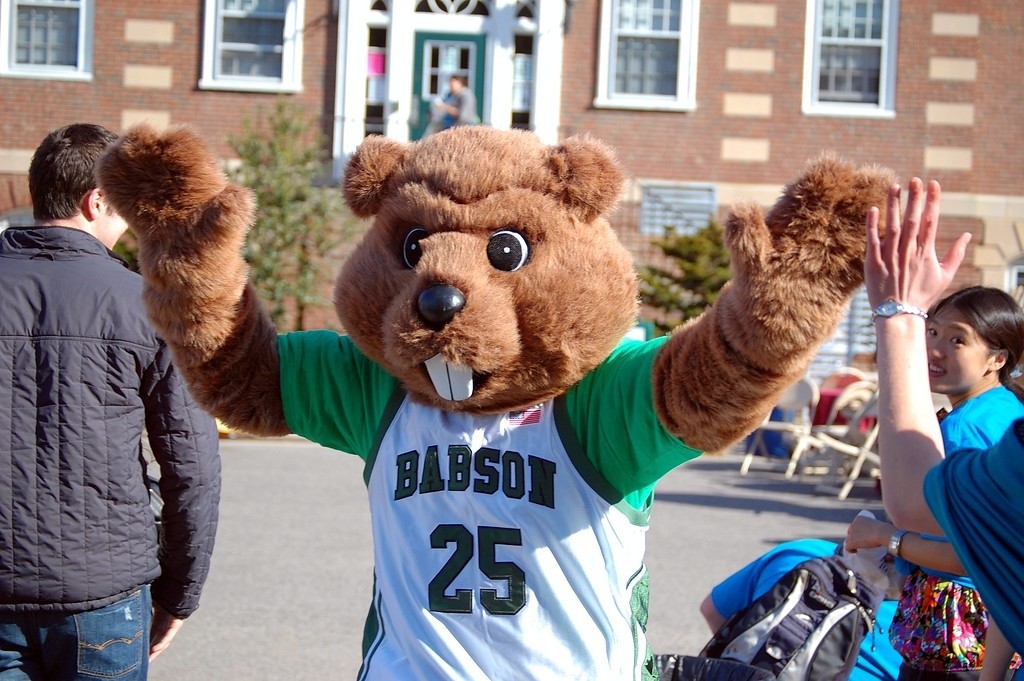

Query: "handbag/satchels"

xmin=888 ymin=567 xmax=1023 ymax=672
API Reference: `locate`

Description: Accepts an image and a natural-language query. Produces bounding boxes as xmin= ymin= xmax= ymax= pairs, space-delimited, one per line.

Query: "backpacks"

xmin=700 ymin=557 xmax=880 ymax=681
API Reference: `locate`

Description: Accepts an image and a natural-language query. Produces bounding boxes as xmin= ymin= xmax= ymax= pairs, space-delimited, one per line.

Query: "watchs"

xmin=869 ymin=296 xmax=930 ymax=321
xmin=887 ymin=529 xmax=907 ymax=559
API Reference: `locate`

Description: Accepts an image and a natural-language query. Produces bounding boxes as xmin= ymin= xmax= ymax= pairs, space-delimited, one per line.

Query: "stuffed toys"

xmin=99 ymin=126 xmax=900 ymax=681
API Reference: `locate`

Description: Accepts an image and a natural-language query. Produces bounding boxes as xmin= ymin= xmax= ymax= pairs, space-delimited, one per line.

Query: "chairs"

xmin=739 ymin=362 xmax=881 ymax=502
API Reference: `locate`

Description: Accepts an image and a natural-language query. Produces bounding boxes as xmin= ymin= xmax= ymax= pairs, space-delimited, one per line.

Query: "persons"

xmin=700 ymin=176 xmax=1024 ymax=681
xmin=434 ymin=75 xmax=476 ymax=126
xmin=0 ymin=124 xmax=222 ymax=681
